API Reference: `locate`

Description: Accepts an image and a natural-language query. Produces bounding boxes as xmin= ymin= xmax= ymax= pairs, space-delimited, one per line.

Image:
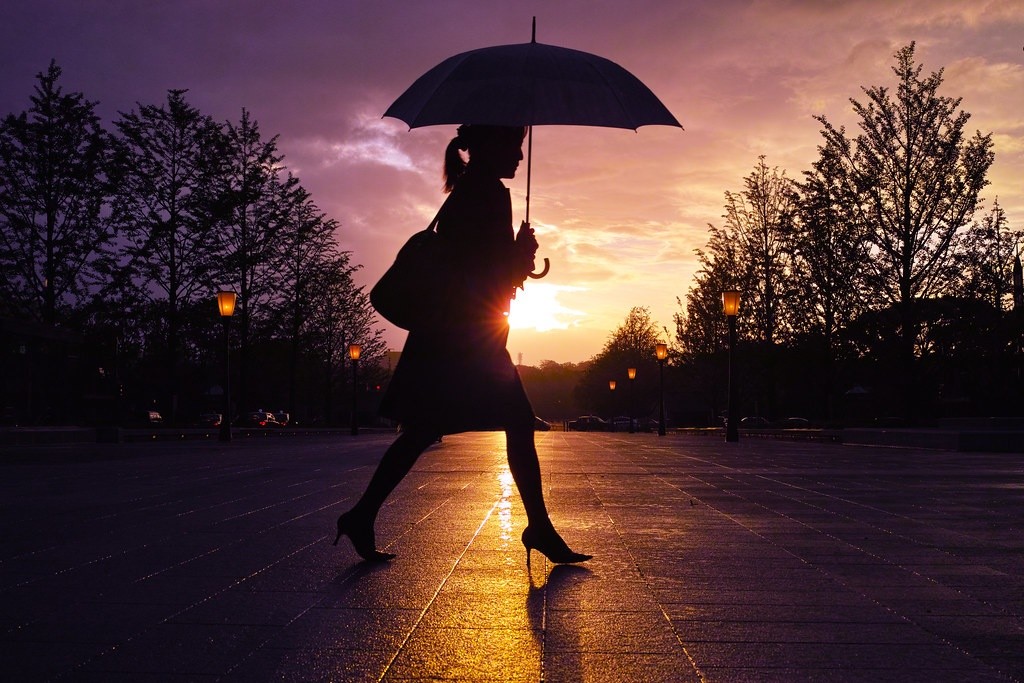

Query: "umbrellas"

xmin=381 ymin=15 xmax=686 ymax=278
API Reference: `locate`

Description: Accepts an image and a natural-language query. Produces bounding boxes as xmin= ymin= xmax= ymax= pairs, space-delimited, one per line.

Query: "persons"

xmin=333 ymin=120 xmax=595 ymax=568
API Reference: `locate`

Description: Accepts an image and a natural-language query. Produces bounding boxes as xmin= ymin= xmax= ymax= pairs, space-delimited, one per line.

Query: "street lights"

xmin=216 ymin=289 xmax=236 ymax=441
xmin=655 ymin=343 xmax=668 ymax=436
xmin=718 ymin=284 xmax=744 ymax=444
xmin=628 ymin=368 xmax=637 ymax=434
xmin=349 ymin=343 xmax=362 ymax=439
xmin=610 ymin=380 xmax=616 ymax=432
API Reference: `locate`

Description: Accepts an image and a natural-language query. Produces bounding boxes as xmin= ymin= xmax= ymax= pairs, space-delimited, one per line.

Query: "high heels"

xmin=331 ymin=507 xmax=396 ymax=562
xmin=521 ymin=526 xmax=593 ymax=566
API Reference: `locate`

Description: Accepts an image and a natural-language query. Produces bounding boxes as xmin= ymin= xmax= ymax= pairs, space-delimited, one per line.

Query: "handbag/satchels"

xmin=368 ymin=188 xmax=474 ymax=335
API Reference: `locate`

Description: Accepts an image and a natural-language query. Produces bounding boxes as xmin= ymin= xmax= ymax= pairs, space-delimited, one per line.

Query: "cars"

xmin=129 ymin=408 xmax=290 ymax=428
xmin=533 ymin=416 xmax=553 ymax=431
xmin=738 ymin=416 xmax=811 ymax=430
xmin=568 ymin=415 xmax=641 ymax=432
